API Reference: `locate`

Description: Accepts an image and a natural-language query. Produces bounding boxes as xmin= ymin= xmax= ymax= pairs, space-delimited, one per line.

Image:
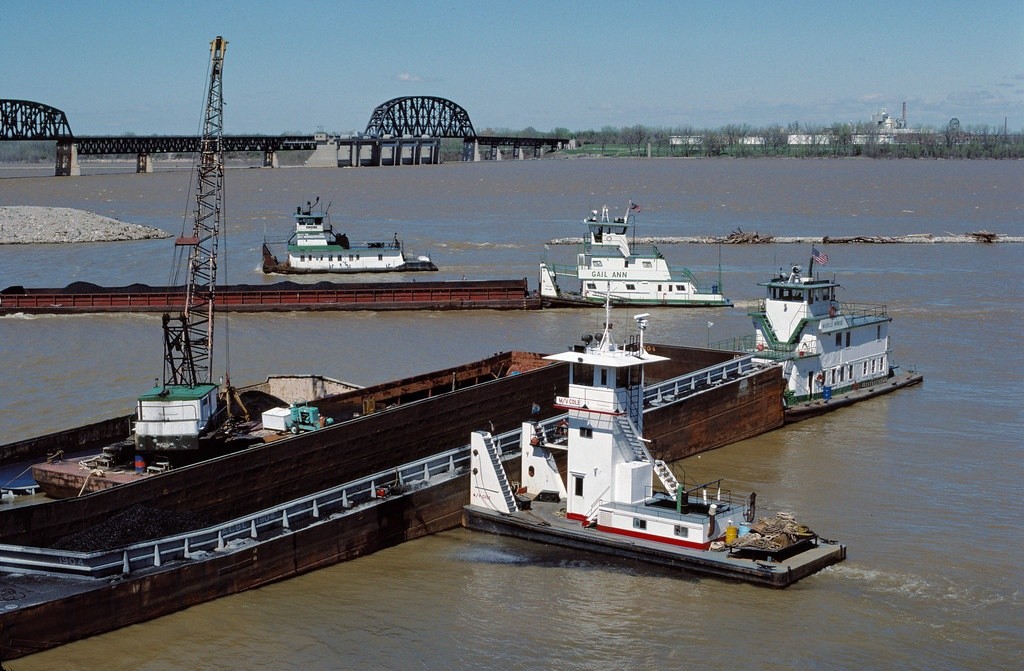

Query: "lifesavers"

xmin=828 ymin=306 xmax=835 ymax=317
xmin=557 ymin=421 xmax=568 ymax=435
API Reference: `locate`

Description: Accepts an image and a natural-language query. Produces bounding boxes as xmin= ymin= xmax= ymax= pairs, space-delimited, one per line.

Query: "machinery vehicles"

xmin=47 ymin=35 xmax=300 ymax=493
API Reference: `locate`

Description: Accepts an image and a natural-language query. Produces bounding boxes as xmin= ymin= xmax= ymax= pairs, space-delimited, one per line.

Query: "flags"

xmin=628 ymin=200 xmax=642 ymax=213
xmin=812 ymin=247 xmax=828 ymax=266
xmin=531 ymin=402 xmax=541 ymax=414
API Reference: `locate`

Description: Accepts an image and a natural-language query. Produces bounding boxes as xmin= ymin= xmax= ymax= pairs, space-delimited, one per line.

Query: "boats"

xmin=461 ymin=280 xmax=846 ymax=589
xmin=708 ymin=242 xmax=924 ymax=424
xmin=260 ymin=195 xmax=442 ymax=272
xmin=532 ymin=198 xmax=735 ymax=309
